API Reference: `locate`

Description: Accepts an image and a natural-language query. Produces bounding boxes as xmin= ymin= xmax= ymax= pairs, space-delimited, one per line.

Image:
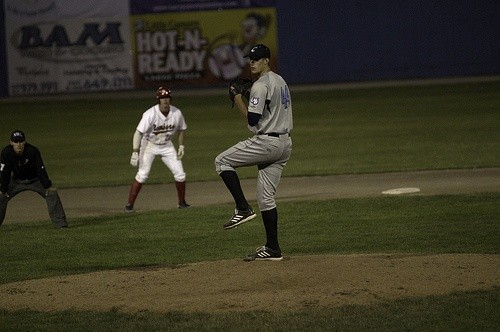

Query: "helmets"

xmin=155 ymin=86 xmax=173 ymax=99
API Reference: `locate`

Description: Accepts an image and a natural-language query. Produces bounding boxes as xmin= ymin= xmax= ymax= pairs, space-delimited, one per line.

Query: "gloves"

xmin=130 ymin=152 xmax=139 ymax=166
xmin=45 ymin=186 xmax=57 ymax=196
xmin=177 ymin=145 xmax=185 ymax=159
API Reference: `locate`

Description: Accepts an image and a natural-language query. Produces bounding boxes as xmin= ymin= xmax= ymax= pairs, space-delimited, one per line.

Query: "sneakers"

xmin=244 ymin=245 xmax=283 ymax=261
xmin=223 ymin=204 xmax=257 ymax=230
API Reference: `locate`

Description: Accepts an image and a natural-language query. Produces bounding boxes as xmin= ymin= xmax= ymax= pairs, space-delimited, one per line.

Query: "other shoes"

xmin=124 ymin=203 xmax=133 ymax=212
xmin=177 ymin=201 xmax=192 ymax=208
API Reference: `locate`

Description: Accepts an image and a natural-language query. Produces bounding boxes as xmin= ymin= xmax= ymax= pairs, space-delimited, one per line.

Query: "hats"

xmin=10 ymin=130 xmax=25 ymax=142
xmin=243 ymin=44 xmax=271 ymax=60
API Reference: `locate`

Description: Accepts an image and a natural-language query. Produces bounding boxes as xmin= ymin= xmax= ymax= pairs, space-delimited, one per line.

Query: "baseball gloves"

xmin=228 ymin=78 xmax=252 ymax=107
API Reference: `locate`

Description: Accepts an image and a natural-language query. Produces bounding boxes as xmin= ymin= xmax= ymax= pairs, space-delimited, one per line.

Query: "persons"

xmin=0 ymin=130 xmax=69 ymax=230
xmin=123 ymin=86 xmax=194 ymax=213
xmin=215 ymin=43 xmax=294 ymax=261
